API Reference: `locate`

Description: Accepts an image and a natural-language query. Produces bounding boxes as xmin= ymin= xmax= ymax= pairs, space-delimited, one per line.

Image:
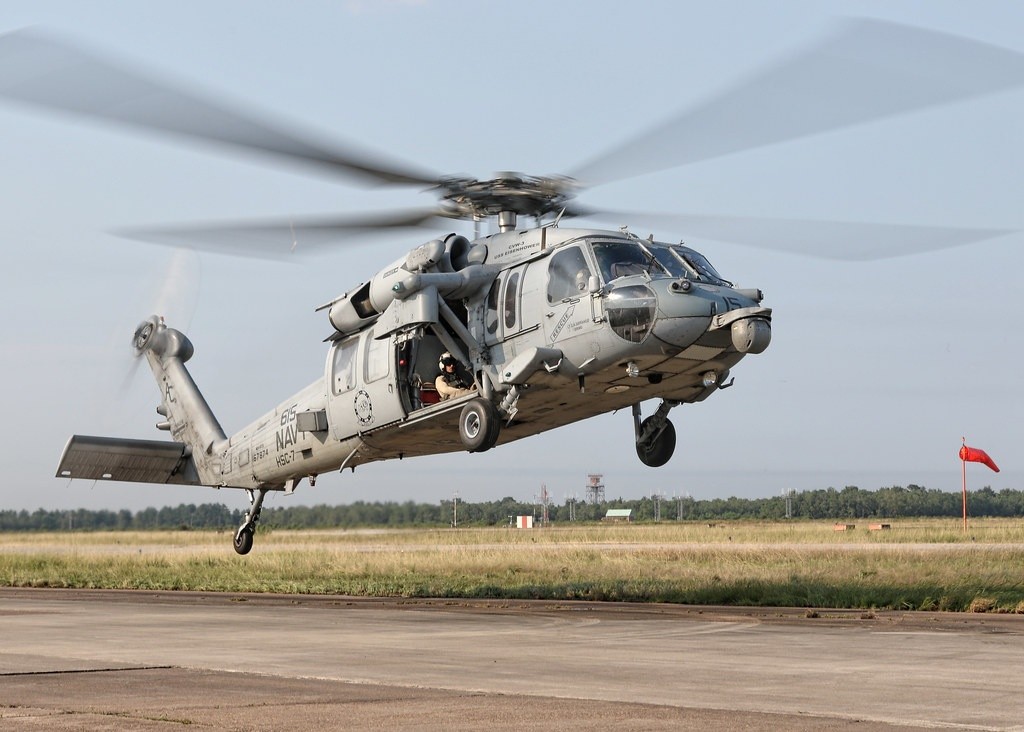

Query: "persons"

xmin=433 ymin=350 xmax=478 ymax=400
xmin=577 ymin=244 xmax=610 ymax=293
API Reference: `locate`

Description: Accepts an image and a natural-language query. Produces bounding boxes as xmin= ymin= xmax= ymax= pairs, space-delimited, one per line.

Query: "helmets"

xmin=438 ymin=351 xmax=456 ymax=373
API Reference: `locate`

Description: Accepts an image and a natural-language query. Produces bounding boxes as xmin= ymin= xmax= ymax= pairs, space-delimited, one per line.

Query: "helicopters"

xmin=0 ymin=15 xmax=1024 ymax=555
